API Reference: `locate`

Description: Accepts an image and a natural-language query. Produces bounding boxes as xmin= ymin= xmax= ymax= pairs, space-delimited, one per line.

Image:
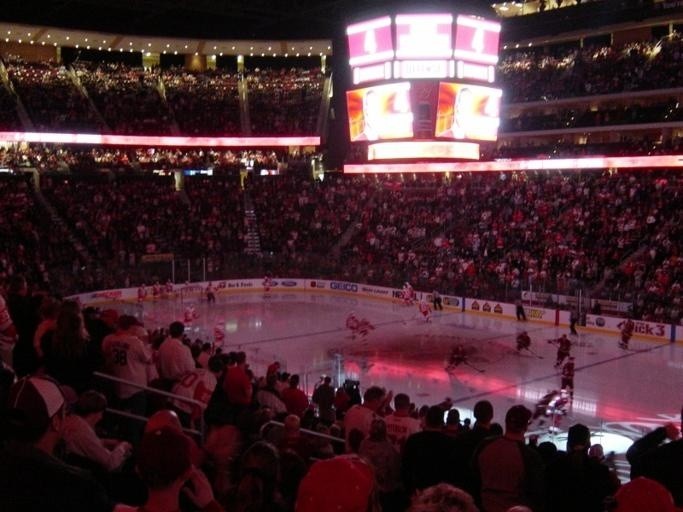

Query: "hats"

xmin=3 ymin=374 xmax=79 ymax=426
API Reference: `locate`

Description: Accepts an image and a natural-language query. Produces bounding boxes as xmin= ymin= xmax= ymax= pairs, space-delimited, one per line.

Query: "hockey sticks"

xmin=526 ymin=348 xmax=545 ymax=359
xmin=459 ymin=359 xmax=486 ymax=372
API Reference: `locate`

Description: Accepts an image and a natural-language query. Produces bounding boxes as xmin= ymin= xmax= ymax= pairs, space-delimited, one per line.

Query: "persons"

xmin=515 ymin=331 xmax=531 ymax=353
xmin=528 ymin=390 xmax=558 ymax=425
xmin=1 ymin=322 xmax=436 ymax=511
xmin=444 ymin=344 xmax=466 ymax=371
xmin=562 ymin=357 xmax=576 ymax=401
xmin=545 ymin=388 xmax=571 ymax=438
xmin=618 ymin=316 xmax=634 ymax=349
xmin=569 ymin=307 xmax=580 ymax=336
xmin=437 ymin=397 xmax=683 ymax=511
xmin=548 ymin=333 xmax=572 ymax=367
xmin=1 ymin=31 xmax=681 ymax=323
